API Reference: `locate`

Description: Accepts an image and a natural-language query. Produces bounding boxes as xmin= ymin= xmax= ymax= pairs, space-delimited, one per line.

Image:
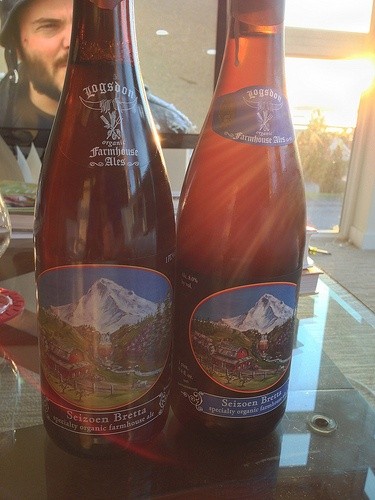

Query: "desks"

xmin=0 ymin=237 xmax=375 ymax=500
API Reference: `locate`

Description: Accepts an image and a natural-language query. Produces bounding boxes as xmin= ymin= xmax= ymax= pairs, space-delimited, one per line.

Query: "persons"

xmin=0 ymin=0 xmax=198 ymax=134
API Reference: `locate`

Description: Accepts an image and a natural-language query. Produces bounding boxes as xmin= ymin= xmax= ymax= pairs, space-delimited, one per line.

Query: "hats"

xmin=0 ymin=0 xmax=29 ymax=48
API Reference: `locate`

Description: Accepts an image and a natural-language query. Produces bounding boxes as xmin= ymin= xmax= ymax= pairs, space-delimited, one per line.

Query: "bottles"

xmin=34 ymin=0 xmax=176 ymax=461
xmin=170 ymin=0 xmax=307 ymax=440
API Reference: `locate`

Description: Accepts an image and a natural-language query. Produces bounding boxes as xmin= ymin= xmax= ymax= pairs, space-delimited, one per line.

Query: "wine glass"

xmin=0 ymin=193 xmax=18 ymax=314
